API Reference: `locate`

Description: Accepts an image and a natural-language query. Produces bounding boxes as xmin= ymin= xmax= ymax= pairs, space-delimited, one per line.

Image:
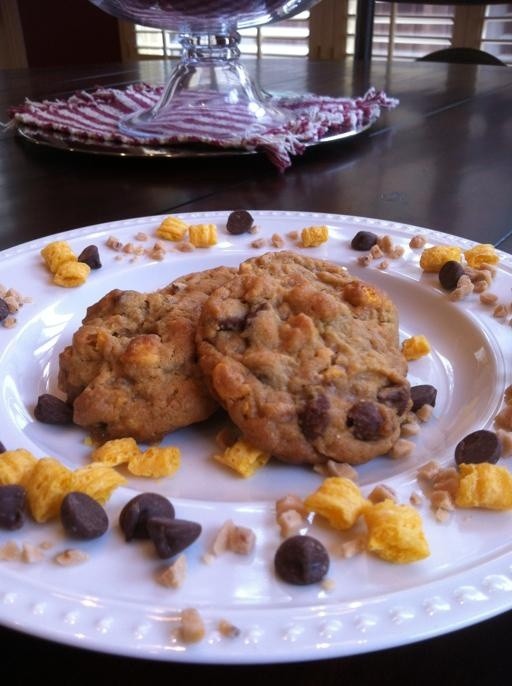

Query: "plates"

xmin=13 ymin=81 xmax=381 ymax=163
xmin=1 ymin=208 xmax=512 ymax=670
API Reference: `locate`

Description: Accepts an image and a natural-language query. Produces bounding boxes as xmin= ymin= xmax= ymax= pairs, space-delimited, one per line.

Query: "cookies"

xmin=194 ymin=250 xmax=411 ymax=462
xmin=57 ymin=265 xmax=242 ymax=446
